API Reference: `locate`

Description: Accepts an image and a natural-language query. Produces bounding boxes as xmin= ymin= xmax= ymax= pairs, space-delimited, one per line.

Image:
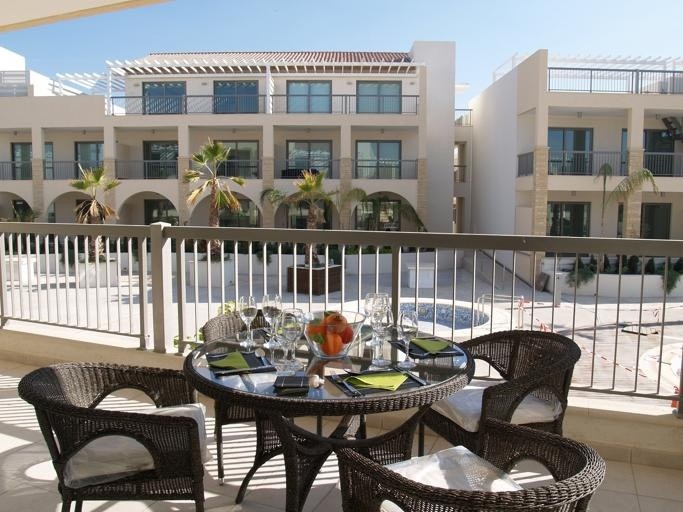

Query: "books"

xmin=273 ymin=375 xmax=309 ymax=397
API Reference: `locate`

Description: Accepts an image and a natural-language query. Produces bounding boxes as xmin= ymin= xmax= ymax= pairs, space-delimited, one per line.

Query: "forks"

xmin=329 ymin=369 xmax=362 ymax=395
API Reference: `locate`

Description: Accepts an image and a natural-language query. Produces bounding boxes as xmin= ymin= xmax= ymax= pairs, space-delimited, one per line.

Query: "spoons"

xmin=343 ymin=368 xmax=393 ymax=376
xmin=255 ymin=346 xmax=268 ymax=366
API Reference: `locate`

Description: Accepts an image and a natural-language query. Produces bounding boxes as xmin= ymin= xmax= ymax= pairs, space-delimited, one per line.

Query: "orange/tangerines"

xmin=308 ymin=311 xmax=354 ymax=355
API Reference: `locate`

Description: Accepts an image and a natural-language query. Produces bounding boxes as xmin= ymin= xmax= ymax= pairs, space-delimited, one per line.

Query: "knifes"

xmin=395 ymin=365 xmax=427 ymax=386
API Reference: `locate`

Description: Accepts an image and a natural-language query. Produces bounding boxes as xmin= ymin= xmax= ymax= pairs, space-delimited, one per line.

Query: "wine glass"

xmin=364 ymin=291 xmax=420 ymax=369
xmin=237 ymin=295 xmax=306 ymax=377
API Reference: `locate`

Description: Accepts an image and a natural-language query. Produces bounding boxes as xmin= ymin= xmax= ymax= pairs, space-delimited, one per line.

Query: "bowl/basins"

xmin=298 ymin=309 xmax=367 ymax=359
